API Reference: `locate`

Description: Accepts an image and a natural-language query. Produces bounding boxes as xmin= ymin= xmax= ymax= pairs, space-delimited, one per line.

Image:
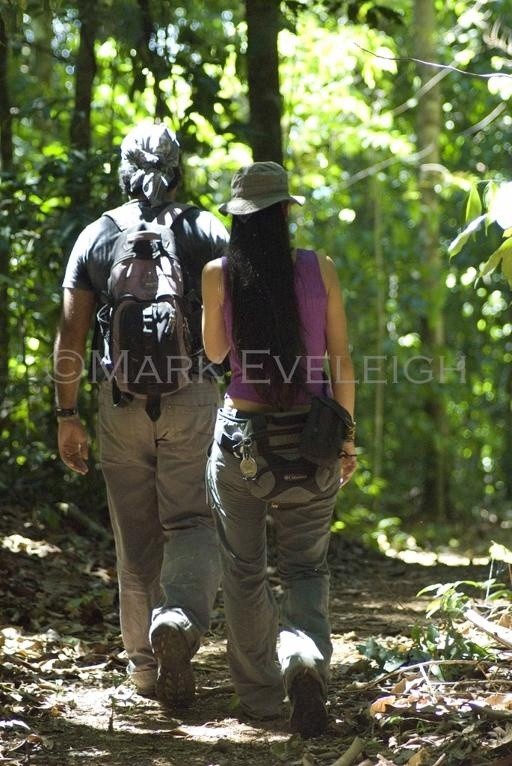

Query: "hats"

xmin=216 ymin=160 xmax=307 ymax=217
xmin=118 ymin=121 xmax=181 ymax=200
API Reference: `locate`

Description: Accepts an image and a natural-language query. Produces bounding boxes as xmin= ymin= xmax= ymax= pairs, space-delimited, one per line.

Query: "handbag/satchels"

xmin=238 ymin=413 xmax=342 ymax=507
xmin=295 ymin=393 xmax=356 ymax=468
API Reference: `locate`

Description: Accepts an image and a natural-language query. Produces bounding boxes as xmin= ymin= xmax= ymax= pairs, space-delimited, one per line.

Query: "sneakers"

xmin=149 ymin=619 xmax=195 ymax=709
xmin=289 ymin=673 xmax=330 ymax=740
xmin=135 ymin=684 xmax=155 ymax=696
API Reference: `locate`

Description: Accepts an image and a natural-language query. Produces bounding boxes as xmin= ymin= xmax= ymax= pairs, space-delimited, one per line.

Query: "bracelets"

xmin=55 ymin=407 xmax=77 ymax=417
xmin=344 ymin=420 xmax=356 ymax=442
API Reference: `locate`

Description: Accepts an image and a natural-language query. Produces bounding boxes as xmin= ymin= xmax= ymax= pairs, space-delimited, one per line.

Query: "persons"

xmin=201 ymin=160 xmax=358 ymax=737
xmin=52 ymin=121 xmax=235 ymax=715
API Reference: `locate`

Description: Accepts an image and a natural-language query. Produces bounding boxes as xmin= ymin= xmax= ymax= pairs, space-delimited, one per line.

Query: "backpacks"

xmin=94 ymin=202 xmax=199 ymax=422
